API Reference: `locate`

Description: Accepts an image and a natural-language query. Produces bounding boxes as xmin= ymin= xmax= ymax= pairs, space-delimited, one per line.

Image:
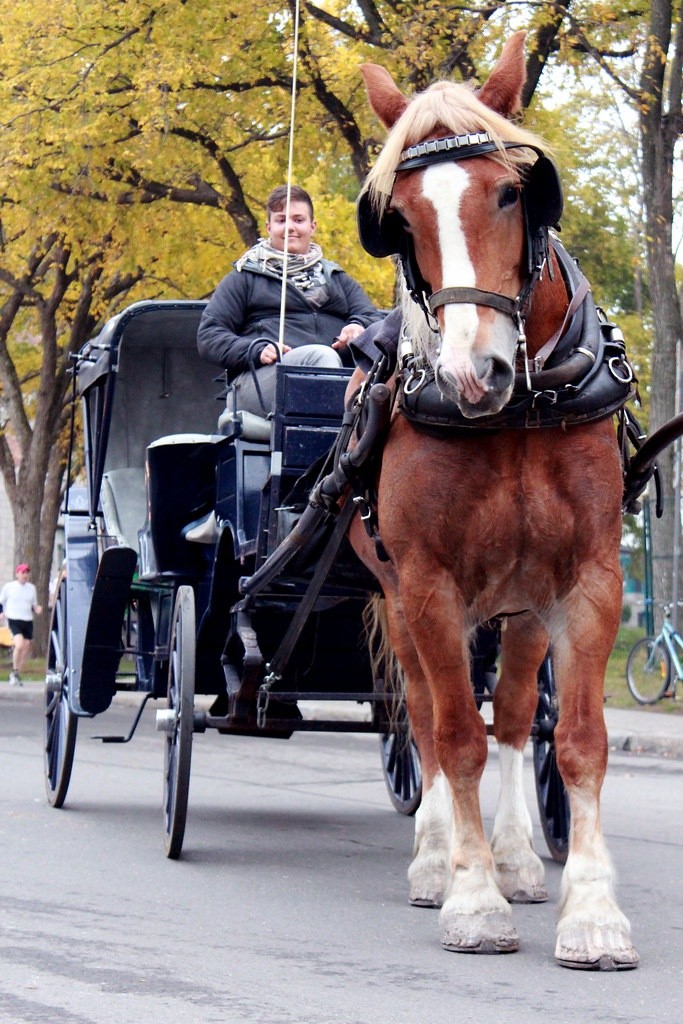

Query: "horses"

xmin=340 ymin=29 xmax=644 ymax=972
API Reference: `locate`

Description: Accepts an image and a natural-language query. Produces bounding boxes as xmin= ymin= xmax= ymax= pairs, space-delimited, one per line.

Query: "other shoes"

xmin=9 ymin=672 xmax=23 ymax=687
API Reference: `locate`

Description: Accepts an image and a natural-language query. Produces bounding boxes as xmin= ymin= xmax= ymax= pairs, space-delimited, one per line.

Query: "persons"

xmin=194 ymin=183 xmax=381 ymax=491
xmin=0 ymin=561 xmax=44 ymax=687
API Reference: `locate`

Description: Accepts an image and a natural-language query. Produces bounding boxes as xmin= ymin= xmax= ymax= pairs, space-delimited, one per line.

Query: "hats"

xmin=17 ymin=564 xmax=30 ymax=574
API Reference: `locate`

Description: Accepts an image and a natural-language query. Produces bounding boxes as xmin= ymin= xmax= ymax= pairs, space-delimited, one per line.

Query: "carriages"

xmin=42 ymin=29 xmax=641 ymax=973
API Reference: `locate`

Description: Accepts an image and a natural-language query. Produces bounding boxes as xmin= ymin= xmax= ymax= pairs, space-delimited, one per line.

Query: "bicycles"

xmin=625 ymin=597 xmax=683 ymax=705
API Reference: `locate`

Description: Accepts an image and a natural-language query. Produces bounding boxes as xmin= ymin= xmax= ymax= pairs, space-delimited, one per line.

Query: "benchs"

xmin=213 ymin=366 xmax=271 ymax=541
xmin=99 ymin=433 xmax=228 ymax=584
